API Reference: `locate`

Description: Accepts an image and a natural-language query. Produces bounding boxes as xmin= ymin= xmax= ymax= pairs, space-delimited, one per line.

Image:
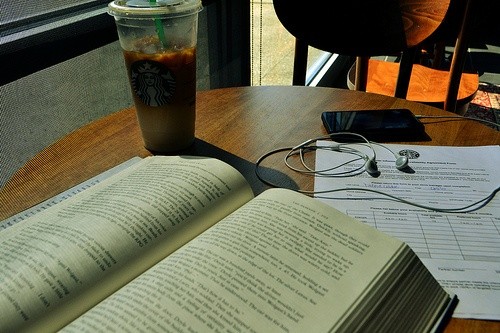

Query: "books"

xmin=0 ymin=154 xmax=459 ymax=333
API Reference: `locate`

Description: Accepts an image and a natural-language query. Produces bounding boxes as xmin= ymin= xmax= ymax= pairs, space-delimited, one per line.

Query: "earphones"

xmin=366 ymin=156 xmax=378 ymax=173
xmin=391 ymin=150 xmax=409 ymax=168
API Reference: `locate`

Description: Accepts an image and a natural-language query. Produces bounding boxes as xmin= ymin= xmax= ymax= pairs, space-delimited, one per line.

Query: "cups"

xmin=108 ymin=0 xmax=205 ymax=155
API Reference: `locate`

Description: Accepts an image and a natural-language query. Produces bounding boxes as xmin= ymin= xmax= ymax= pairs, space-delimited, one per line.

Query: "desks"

xmin=1 ymin=85 xmax=500 ymax=333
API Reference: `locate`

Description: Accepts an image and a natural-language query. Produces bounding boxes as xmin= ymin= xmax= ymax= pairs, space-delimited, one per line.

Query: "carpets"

xmin=452 ymin=81 xmax=499 ymax=132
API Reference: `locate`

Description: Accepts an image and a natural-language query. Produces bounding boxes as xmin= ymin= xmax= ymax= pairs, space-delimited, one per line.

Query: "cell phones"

xmin=321 ymin=109 xmax=425 ymax=143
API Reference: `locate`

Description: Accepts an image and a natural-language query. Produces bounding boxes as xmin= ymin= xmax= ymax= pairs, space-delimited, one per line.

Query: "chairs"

xmin=271 ymin=0 xmax=480 ymax=120
